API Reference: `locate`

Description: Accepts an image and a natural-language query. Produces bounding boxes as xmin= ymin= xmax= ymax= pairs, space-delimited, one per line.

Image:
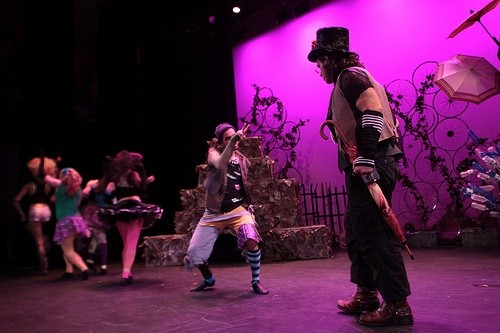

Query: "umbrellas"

xmin=434 ymin=54 xmax=500 ymax=105
xmin=319 ymin=120 xmax=414 ymax=261
xmin=447 ymin=0 xmax=500 ymax=46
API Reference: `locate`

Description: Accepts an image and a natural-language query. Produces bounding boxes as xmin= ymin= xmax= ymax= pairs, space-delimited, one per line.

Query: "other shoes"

xmin=189 ymin=279 xmax=216 ymax=291
xmin=100 ymin=267 xmax=108 ymax=275
xmin=56 ymin=271 xmax=74 ymax=280
xmin=77 ymin=269 xmax=89 ymax=281
xmin=254 ymin=281 xmax=269 ymax=295
xmin=120 ymin=276 xmax=133 ymax=287
xmin=83 ymin=260 xmax=98 ymax=273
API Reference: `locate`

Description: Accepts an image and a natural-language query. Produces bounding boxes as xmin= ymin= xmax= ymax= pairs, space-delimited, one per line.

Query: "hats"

xmin=305 ymin=27 xmax=359 ymax=57
xmin=215 ymin=123 xmax=236 ymax=142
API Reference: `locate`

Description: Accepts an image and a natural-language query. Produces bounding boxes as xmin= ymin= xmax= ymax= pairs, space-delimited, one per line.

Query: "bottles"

xmin=459 ymin=130 xmax=500 ymax=217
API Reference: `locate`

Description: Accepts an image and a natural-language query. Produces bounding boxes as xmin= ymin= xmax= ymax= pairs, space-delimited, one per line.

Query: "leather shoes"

xmin=336 ymin=285 xmax=380 ymax=311
xmin=358 ymin=298 xmax=414 ymax=326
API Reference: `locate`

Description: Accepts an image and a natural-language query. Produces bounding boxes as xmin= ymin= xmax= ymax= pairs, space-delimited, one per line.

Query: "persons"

xmin=13 ymin=151 xmax=163 ymax=286
xmin=308 ymin=27 xmax=414 ymax=326
xmin=187 ymin=122 xmax=269 ymax=295
